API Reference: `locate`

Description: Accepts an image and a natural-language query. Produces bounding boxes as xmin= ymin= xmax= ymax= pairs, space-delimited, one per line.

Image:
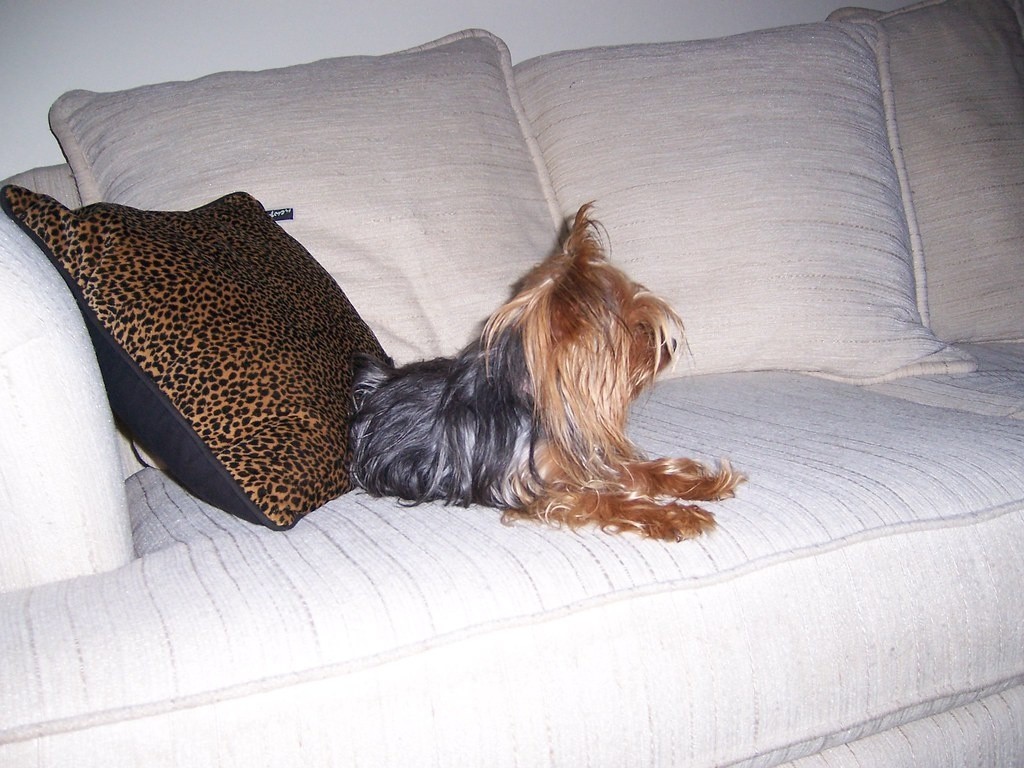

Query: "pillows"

xmin=513 ymin=18 xmax=980 ymax=386
xmin=48 ymin=28 xmax=568 ymax=370
xmin=1 ymin=184 xmax=393 ymax=533
xmin=827 ymin=1 xmax=1024 ymax=344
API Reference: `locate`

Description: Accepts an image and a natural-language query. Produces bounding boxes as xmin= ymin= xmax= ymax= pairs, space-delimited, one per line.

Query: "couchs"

xmin=0 ymin=163 xmax=1024 ymax=768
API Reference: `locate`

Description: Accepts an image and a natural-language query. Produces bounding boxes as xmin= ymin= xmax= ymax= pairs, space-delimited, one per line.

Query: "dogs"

xmin=342 ymin=201 xmax=747 ymax=542
